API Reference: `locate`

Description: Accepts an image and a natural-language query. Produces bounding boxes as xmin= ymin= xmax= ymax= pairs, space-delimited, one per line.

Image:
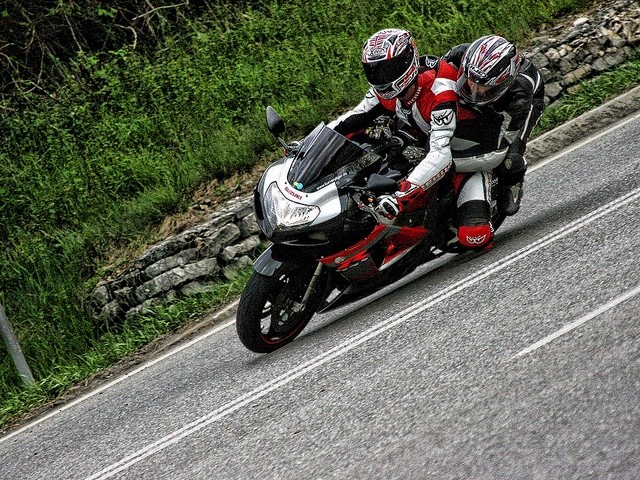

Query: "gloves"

xmin=374 ymin=195 xmax=400 ymax=226
xmin=284 ymin=140 xmax=301 ymax=157
xmin=365 ymin=115 xmax=393 ymax=140
xmin=403 ymin=145 xmax=425 ymax=165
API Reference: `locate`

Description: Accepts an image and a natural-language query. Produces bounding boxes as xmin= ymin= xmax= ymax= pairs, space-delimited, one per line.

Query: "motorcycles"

xmin=236 ymin=104 xmax=524 ymax=353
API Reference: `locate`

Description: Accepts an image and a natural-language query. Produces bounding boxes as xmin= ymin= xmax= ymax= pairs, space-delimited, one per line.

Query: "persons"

xmin=439 ymin=33 xmax=545 ymax=188
xmin=326 ymin=28 xmax=495 ymax=252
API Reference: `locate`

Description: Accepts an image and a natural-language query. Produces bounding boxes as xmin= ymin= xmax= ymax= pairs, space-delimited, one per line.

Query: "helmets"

xmin=361 ymin=28 xmax=420 ymax=100
xmin=457 ymin=34 xmax=517 ymax=106
